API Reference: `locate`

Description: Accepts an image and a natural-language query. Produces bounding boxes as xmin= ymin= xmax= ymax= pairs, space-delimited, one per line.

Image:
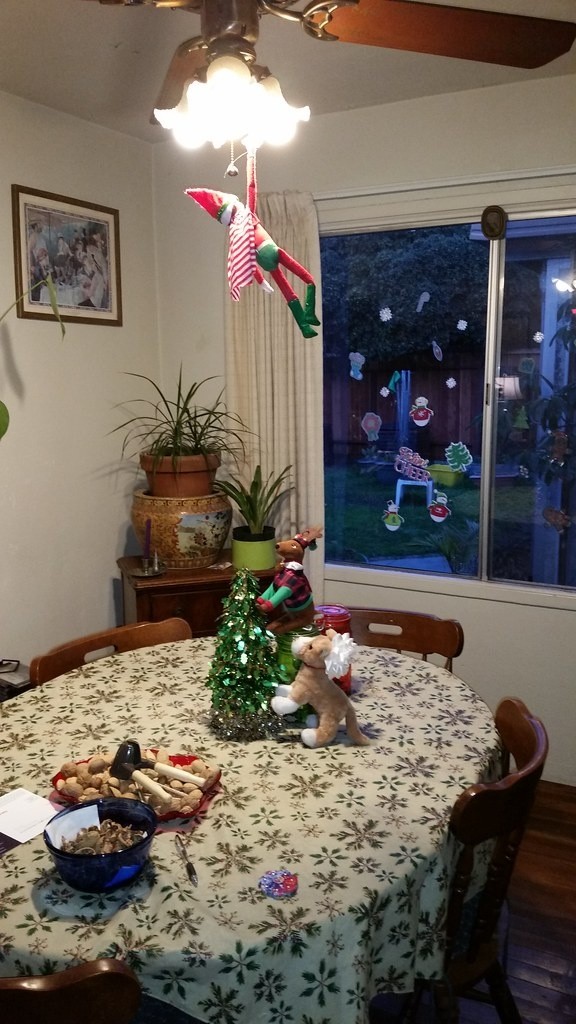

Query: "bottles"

xmin=275 ymin=622 xmax=324 ymax=684
xmin=313 ymin=602 xmax=352 ymax=699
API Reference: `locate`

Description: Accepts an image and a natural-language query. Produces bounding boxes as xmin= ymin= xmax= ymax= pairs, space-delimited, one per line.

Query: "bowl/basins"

xmin=42 ymin=796 xmax=157 ymax=893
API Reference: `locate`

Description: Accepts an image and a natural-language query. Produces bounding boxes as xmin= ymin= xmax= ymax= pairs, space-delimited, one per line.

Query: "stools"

xmin=394 ymin=476 xmax=435 ymax=512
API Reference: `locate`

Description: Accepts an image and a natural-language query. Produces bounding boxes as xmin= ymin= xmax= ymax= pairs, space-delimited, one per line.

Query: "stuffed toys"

xmin=182 ymin=147 xmax=321 ymax=338
xmin=271 ymin=628 xmax=374 ymax=749
xmin=254 ymin=526 xmax=325 ymax=635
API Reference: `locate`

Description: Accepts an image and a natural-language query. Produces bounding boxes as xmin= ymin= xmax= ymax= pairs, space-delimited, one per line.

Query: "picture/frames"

xmin=11 ymin=183 xmax=123 ymax=327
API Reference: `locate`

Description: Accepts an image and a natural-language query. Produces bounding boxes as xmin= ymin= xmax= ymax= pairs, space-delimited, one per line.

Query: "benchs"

xmin=361 ymin=424 xmax=429 ymax=476
xmin=467 ymin=462 xmax=518 ymax=488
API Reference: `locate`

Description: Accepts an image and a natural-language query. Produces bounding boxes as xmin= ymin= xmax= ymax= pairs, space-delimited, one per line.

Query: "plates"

xmin=51 ymin=752 xmax=214 ymax=825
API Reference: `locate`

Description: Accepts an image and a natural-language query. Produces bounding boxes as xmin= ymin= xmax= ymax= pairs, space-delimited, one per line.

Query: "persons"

xmin=28 ymin=214 xmax=107 ymax=300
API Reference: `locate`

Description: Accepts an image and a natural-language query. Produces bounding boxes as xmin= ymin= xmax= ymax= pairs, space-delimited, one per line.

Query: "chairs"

xmin=29 ymin=614 xmax=193 ymax=687
xmin=409 ymin=696 xmax=550 ymax=1023
xmin=344 ymin=607 xmax=465 ymax=675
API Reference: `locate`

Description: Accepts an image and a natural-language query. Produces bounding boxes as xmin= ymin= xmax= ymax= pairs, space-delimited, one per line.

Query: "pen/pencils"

xmin=173 ymin=834 xmax=200 ymax=885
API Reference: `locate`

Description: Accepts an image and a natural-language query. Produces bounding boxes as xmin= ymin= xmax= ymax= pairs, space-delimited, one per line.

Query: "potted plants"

xmin=106 ymin=369 xmax=246 ymax=569
xmin=208 ymin=462 xmax=298 ymax=574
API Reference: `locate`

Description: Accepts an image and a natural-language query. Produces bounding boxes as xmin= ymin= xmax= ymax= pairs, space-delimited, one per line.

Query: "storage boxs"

xmin=427 ymin=463 xmax=464 ymax=488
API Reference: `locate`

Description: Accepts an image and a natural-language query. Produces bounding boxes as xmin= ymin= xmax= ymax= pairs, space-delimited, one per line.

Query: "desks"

xmin=116 ymin=548 xmax=276 ymax=637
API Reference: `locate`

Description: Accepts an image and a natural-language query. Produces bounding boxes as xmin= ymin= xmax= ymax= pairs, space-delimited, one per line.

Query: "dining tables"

xmin=0 ymin=957 xmax=141 ymax=1024
xmin=0 ymin=633 xmax=498 ymax=1024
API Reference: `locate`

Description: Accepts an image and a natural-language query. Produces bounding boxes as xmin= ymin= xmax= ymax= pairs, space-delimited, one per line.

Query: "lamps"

xmin=98 ymin=0 xmax=361 ymax=181
xmin=550 ymin=266 xmax=576 ymax=291
